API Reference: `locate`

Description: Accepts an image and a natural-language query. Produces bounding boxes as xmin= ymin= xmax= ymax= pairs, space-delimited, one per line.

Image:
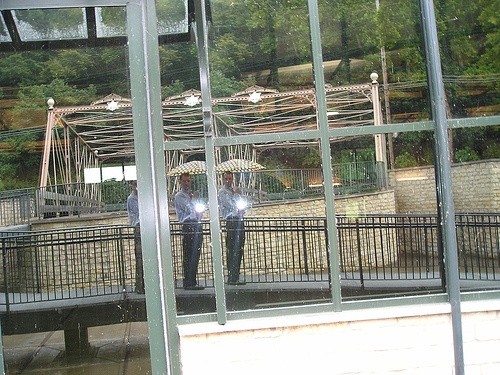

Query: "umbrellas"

xmin=215 ymin=158 xmax=266 ymax=174
xmin=166 ymin=160 xmax=206 ymax=177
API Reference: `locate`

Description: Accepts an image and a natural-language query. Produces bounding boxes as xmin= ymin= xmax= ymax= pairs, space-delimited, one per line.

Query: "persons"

xmin=174 ymin=172 xmax=204 ymax=291
xmin=126 ymin=179 xmax=146 ymax=295
xmin=218 ymin=170 xmax=249 ymax=286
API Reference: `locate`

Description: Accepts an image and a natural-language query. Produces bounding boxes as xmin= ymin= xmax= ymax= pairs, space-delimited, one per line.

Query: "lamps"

xmin=370 ymin=73 xmax=378 ymax=84
xmin=47 ymin=99 xmax=54 ymax=110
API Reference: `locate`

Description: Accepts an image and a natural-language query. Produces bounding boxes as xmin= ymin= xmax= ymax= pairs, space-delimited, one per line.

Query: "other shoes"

xmin=182 ymin=285 xmax=205 ymax=290
xmin=134 ymin=288 xmax=144 ymax=294
xmin=229 ymin=280 xmax=246 ymax=285
